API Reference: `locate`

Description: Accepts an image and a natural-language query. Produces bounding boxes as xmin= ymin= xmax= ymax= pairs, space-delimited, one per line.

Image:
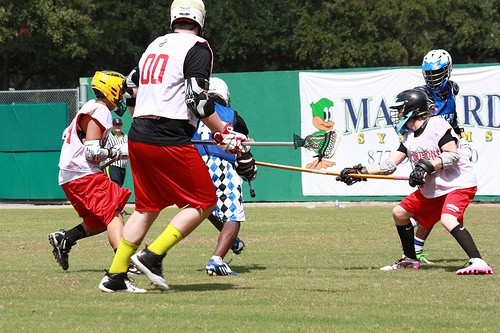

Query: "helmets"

xmin=422 ymin=49 xmax=453 ymax=80
xmin=91 ymin=71 xmax=127 ymax=106
xmin=170 ymin=0 xmax=206 ymax=31
xmin=389 ymin=90 xmax=428 ymax=120
xmin=208 ymin=77 xmax=231 ymax=106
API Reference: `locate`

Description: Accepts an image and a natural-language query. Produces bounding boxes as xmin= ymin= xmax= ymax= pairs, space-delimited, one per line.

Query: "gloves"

xmin=409 ymin=158 xmax=436 ymax=187
xmin=212 ymin=123 xmax=250 ymax=154
xmin=237 ymin=153 xmax=257 ymax=180
xmin=336 ymin=163 xmax=367 ymax=186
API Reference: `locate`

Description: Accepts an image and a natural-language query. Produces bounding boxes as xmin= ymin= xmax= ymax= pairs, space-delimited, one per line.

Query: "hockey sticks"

xmin=192 ymin=132 xmax=340 ymax=157
xmin=254 ymin=161 xmax=428 ymax=186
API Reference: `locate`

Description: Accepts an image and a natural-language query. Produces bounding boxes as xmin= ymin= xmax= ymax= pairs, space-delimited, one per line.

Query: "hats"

xmin=113 ymin=118 xmax=122 ymax=125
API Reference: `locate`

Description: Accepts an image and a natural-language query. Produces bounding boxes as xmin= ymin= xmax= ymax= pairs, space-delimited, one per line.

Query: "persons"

xmin=396 ymin=49 xmax=462 ymax=265
xmin=98 ymin=0 xmax=254 ymax=295
xmin=336 ymin=89 xmax=494 ymax=275
xmin=104 ymin=118 xmax=132 ymax=216
xmin=190 ymin=77 xmax=257 ymax=276
xmin=48 ymin=70 xmax=145 ymax=275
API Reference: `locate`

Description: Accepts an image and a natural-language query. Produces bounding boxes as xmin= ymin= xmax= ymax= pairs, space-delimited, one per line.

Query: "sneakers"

xmin=99 ymin=269 xmax=146 ymax=293
xmin=380 ymin=254 xmax=420 ymax=271
xmin=456 ymin=257 xmax=493 ymax=275
xmin=232 ymin=239 xmax=245 ymax=254
xmin=206 ymin=258 xmax=238 ymax=276
xmin=48 ymin=229 xmax=77 ymax=270
xmin=126 ymin=263 xmax=144 ymax=275
xmin=130 ymin=244 xmax=170 ymax=291
